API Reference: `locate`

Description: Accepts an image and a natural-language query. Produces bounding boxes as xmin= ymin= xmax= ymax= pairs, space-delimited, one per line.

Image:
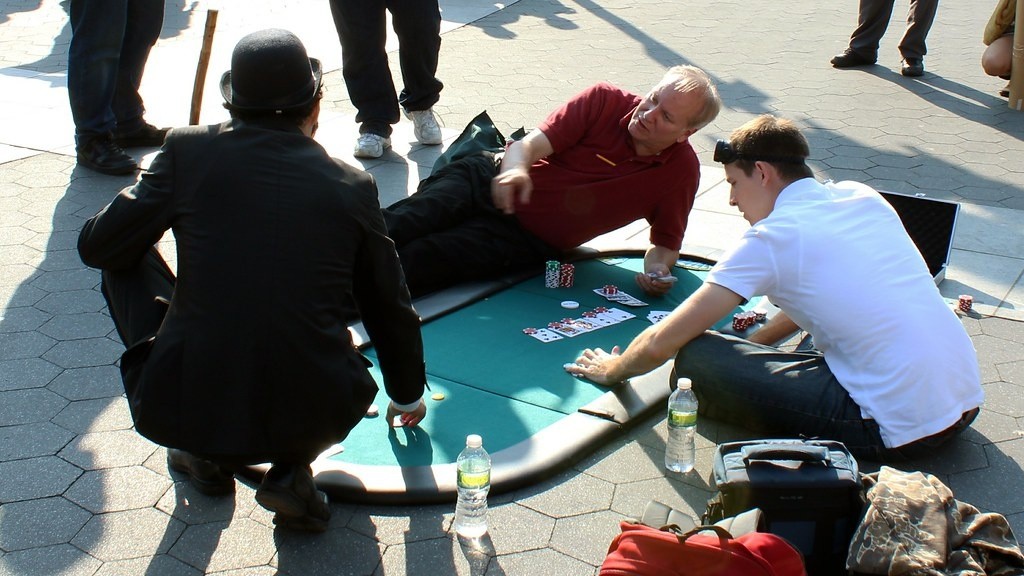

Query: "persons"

xmin=74 ymin=28 xmax=430 ymax=534
xmin=67 ymin=0 xmax=172 ymax=175
xmin=830 ymin=0 xmax=939 ymax=77
xmin=329 ymin=0 xmax=444 ymax=159
xmin=562 ymin=116 xmax=990 ymax=459
xmin=981 ymin=0 xmax=1024 ymax=111
xmin=348 ymin=66 xmax=720 ymax=326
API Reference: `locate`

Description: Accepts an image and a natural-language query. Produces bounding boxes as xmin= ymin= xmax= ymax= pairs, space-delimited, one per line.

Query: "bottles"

xmin=455 ymin=434 xmax=492 ymax=539
xmin=664 ymin=378 xmax=699 ymax=473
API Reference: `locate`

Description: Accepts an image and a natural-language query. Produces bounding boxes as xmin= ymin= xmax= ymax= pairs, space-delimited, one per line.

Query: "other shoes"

xmin=999 ymin=79 xmax=1011 ymax=98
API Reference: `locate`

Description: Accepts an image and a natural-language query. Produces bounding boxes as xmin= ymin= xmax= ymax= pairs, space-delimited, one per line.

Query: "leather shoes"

xmin=167 ymin=444 xmax=236 ymax=495
xmin=255 ymin=462 xmax=332 ymax=530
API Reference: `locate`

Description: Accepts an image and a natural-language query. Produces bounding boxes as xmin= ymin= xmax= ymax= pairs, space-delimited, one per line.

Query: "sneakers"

xmin=353 ymin=133 xmax=391 ymax=158
xmin=901 ymin=56 xmax=923 ymax=77
xmin=403 ymin=105 xmax=445 ymax=145
xmin=831 ymin=47 xmax=877 ymax=67
xmin=76 ymin=143 xmax=137 ymax=176
xmin=113 ymin=119 xmax=172 ymax=147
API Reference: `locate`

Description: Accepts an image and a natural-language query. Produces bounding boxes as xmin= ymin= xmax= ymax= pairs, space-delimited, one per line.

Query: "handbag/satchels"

xmin=602 ymin=519 xmax=807 ymax=576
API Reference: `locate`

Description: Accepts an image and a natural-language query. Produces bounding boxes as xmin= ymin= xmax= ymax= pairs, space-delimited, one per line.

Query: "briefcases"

xmin=714 ymin=438 xmax=867 ymax=576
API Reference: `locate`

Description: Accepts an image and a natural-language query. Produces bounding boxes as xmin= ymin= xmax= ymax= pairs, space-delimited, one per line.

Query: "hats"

xmin=221 ymin=29 xmax=322 ymax=114
